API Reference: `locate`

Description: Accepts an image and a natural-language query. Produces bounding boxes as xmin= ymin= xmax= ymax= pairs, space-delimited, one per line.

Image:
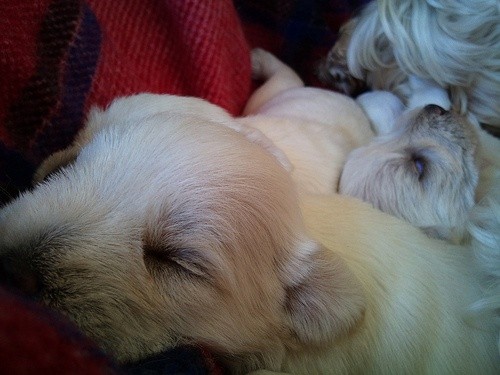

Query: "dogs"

xmin=345 ymin=0 xmax=500 ymax=128
xmin=338 ymin=72 xmax=500 ymax=245
xmin=0 ymin=90 xmax=500 ymax=375
